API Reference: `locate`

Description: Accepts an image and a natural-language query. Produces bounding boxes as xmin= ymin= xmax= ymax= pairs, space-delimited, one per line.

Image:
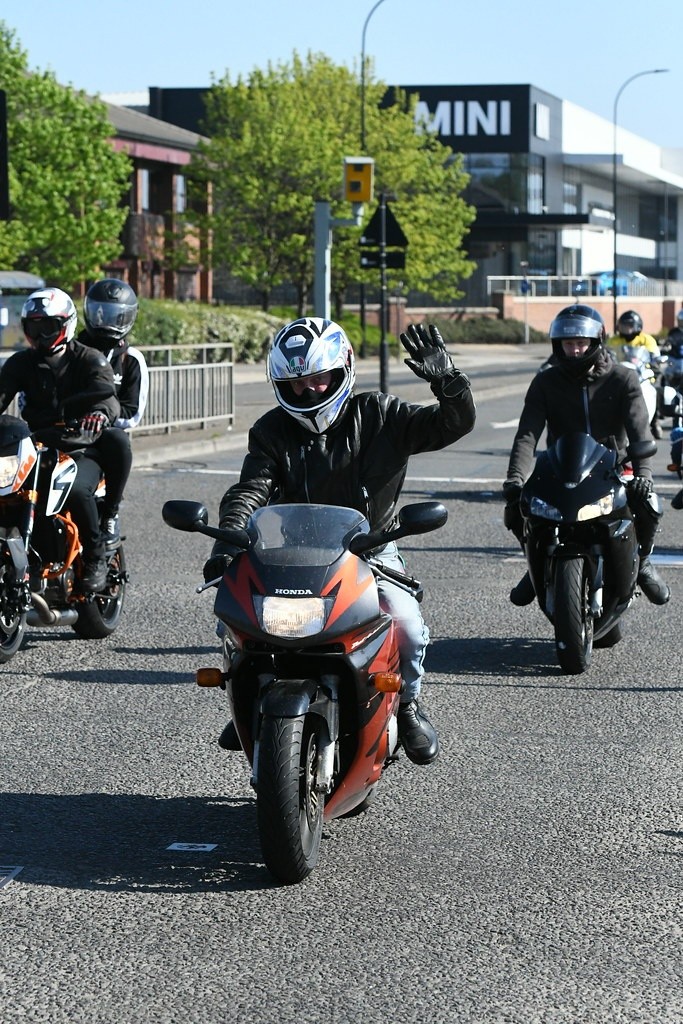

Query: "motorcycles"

xmin=162 ymin=496 xmax=451 ymax=881
xmin=503 ymin=429 xmax=664 ymax=673
xmin=0 ymin=410 xmax=128 ymax=660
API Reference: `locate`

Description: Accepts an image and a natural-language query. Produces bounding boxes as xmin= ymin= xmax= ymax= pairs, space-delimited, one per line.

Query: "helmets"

xmin=266 ymin=316 xmax=356 ymax=435
xmin=677 ymin=309 xmax=683 ymax=331
xmin=82 ymin=278 xmax=138 ymax=347
xmin=549 ymin=304 xmax=606 ymax=372
xmin=21 ymin=287 xmax=78 ymax=357
xmin=617 ymin=310 xmax=643 ymax=342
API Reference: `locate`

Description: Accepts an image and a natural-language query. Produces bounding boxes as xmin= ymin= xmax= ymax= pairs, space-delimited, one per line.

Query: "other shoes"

xmin=650 ymin=420 xmax=663 ymax=439
xmin=671 ymin=487 xmax=683 ymax=510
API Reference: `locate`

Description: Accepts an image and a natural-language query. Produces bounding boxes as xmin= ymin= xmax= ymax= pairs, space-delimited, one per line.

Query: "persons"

xmin=19 ymin=279 xmax=148 ymax=557
xmin=609 ymin=310 xmax=664 ymax=440
xmin=0 ymin=288 xmax=122 ymax=592
xmin=202 ymin=316 xmax=477 ymax=766
xmin=661 ymin=310 xmax=683 ymax=472
xmin=502 ymin=305 xmax=671 ymax=607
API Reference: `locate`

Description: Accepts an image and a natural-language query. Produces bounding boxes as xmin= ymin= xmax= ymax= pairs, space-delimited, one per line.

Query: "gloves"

xmin=80 ymin=410 xmax=109 ymax=434
xmin=400 ymin=323 xmax=471 ymax=400
xmin=203 ymin=552 xmax=234 ymax=589
xmin=502 ymin=476 xmax=523 ymax=502
xmin=629 ymin=476 xmax=653 ymax=500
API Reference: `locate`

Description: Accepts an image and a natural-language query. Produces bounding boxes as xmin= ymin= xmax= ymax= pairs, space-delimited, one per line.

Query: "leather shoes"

xmin=510 ymin=570 xmax=536 ymax=607
xmin=397 ymin=698 xmax=439 ymax=766
xmin=637 ymin=559 xmax=672 ymax=605
xmin=218 ymin=718 xmax=243 ymax=752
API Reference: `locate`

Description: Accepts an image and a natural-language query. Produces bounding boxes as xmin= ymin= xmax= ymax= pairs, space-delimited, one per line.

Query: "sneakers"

xmin=80 ymin=554 xmax=108 ymax=593
xmin=99 ymin=514 xmax=121 ymax=549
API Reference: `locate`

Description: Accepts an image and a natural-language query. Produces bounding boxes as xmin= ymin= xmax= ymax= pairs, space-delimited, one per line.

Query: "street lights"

xmin=610 ymin=66 xmax=671 ymax=332
